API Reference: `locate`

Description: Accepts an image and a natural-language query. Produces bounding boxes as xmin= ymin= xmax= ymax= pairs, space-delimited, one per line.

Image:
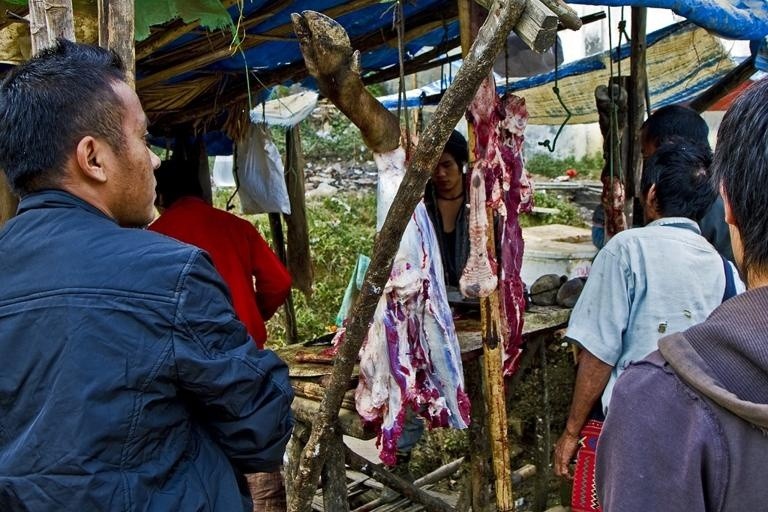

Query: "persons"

xmin=553 ymin=130 xmax=746 ymax=480
xmin=639 ymin=102 xmax=749 ymax=283
xmin=145 ymin=157 xmax=292 ymax=350
xmin=377 ymin=127 xmax=501 ymax=473
xmin=596 ymin=75 xmax=768 ymax=512
xmin=590 ymin=161 xmax=620 ymax=251
xmin=0 ymin=35 xmax=296 ymax=512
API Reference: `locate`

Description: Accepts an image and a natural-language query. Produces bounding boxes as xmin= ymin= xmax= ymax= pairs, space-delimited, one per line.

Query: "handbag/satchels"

xmin=570 ymin=420 xmax=604 ymax=512
